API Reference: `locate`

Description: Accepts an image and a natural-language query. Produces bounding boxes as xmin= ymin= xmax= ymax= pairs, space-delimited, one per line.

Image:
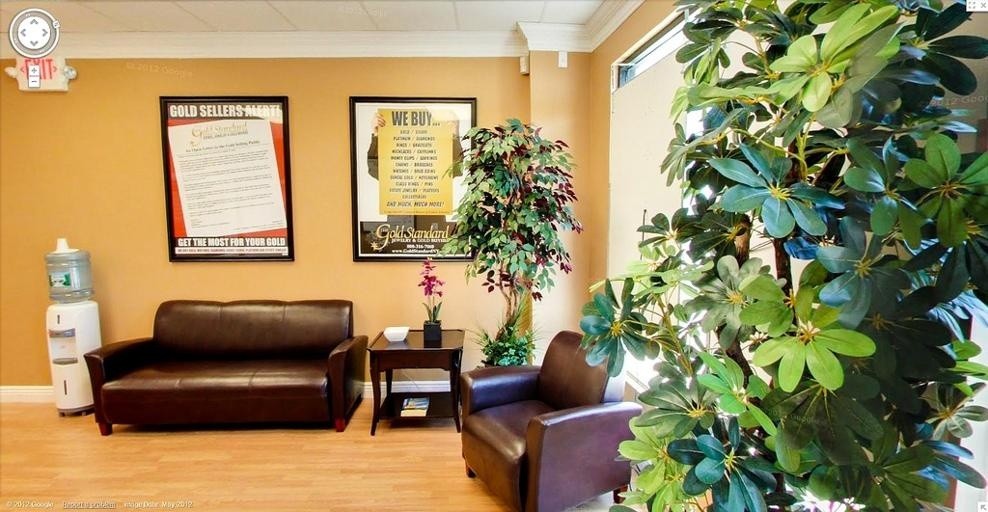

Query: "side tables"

xmin=365 ymin=328 xmax=466 ymax=436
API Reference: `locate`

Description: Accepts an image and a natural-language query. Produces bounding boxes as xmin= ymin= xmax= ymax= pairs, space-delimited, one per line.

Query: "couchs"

xmin=82 ymin=298 xmax=370 ymax=437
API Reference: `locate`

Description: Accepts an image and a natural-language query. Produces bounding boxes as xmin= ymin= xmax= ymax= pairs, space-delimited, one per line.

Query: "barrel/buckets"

xmin=44 ymin=250 xmax=96 ymax=303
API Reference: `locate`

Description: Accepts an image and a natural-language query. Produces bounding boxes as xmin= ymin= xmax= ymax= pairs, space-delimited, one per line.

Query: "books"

xmin=400 ymin=396 xmax=430 ymax=418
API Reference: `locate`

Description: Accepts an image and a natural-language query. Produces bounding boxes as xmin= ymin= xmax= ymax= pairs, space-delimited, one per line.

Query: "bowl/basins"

xmin=383 ymin=326 xmax=410 ymax=342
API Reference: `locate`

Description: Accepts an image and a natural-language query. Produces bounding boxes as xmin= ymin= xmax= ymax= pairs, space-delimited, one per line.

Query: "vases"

xmin=423 ymin=317 xmax=443 ymax=343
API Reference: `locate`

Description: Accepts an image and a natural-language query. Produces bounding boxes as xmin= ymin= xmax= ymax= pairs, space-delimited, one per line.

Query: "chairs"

xmin=459 ymin=328 xmax=643 ymax=512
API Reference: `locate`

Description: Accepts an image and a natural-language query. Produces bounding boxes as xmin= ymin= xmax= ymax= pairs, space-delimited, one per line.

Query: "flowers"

xmin=415 ymin=255 xmax=449 ymax=322
